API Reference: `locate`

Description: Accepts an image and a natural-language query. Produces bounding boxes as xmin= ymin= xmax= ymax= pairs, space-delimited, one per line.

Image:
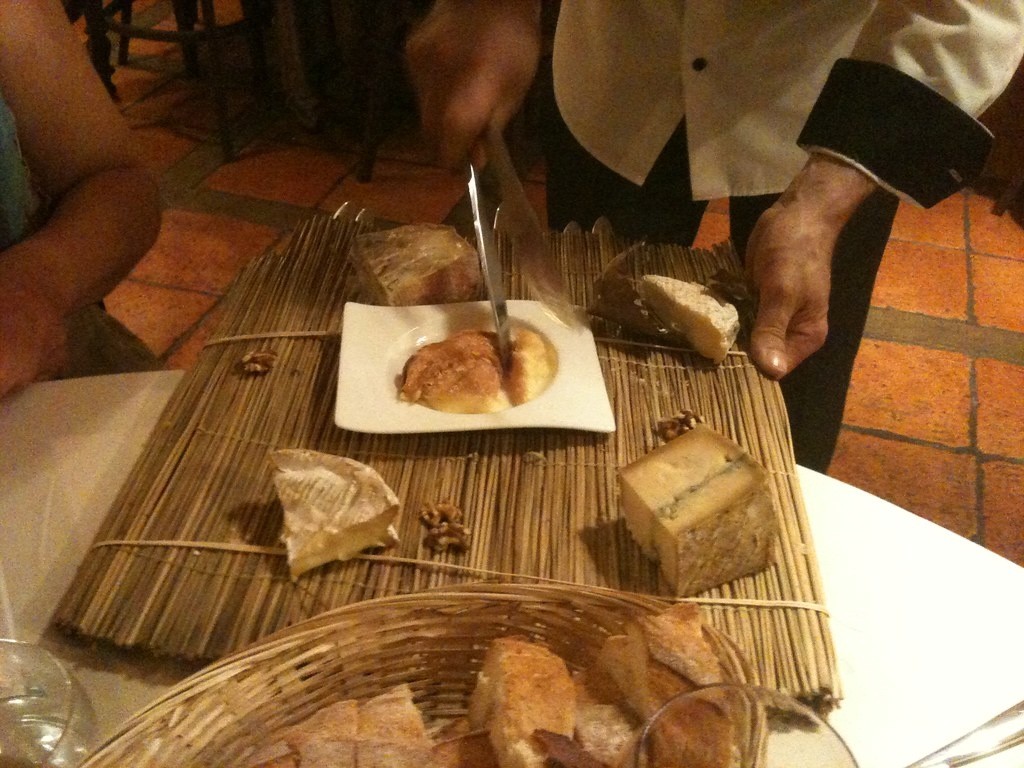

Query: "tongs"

xmin=461 ymin=120 xmax=581 ymax=375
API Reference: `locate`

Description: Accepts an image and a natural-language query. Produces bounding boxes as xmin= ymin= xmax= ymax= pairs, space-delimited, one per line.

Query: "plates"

xmin=332 ymin=301 xmax=615 ymax=436
xmin=600 ymin=243 xmax=757 ymax=343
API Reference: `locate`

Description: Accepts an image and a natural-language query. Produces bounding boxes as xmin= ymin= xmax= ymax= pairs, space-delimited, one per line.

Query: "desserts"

xmin=641 ymin=274 xmax=742 ymax=368
xmin=351 ymin=220 xmax=486 ymax=307
xmin=617 ymin=424 xmax=774 ymax=599
xmin=270 ymin=449 xmax=403 ymax=579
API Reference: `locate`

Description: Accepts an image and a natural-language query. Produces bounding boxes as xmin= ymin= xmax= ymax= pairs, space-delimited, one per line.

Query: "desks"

xmin=1 ymin=371 xmax=1024 ymax=767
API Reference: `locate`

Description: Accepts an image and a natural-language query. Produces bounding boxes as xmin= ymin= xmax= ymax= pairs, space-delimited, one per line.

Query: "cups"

xmin=0 ymin=638 xmax=97 ymax=766
xmin=633 ymin=683 xmax=859 ymax=768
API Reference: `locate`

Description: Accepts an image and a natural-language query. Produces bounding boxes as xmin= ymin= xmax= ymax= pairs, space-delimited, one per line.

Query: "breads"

xmin=229 ymin=600 xmax=743 ymax=768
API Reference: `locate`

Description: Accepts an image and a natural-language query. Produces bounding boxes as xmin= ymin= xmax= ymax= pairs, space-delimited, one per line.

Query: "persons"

xmin=405 ymin=1 xmax=1024 ymax=472
xmin=0 ymin=0 xmax=161 ymax=399
